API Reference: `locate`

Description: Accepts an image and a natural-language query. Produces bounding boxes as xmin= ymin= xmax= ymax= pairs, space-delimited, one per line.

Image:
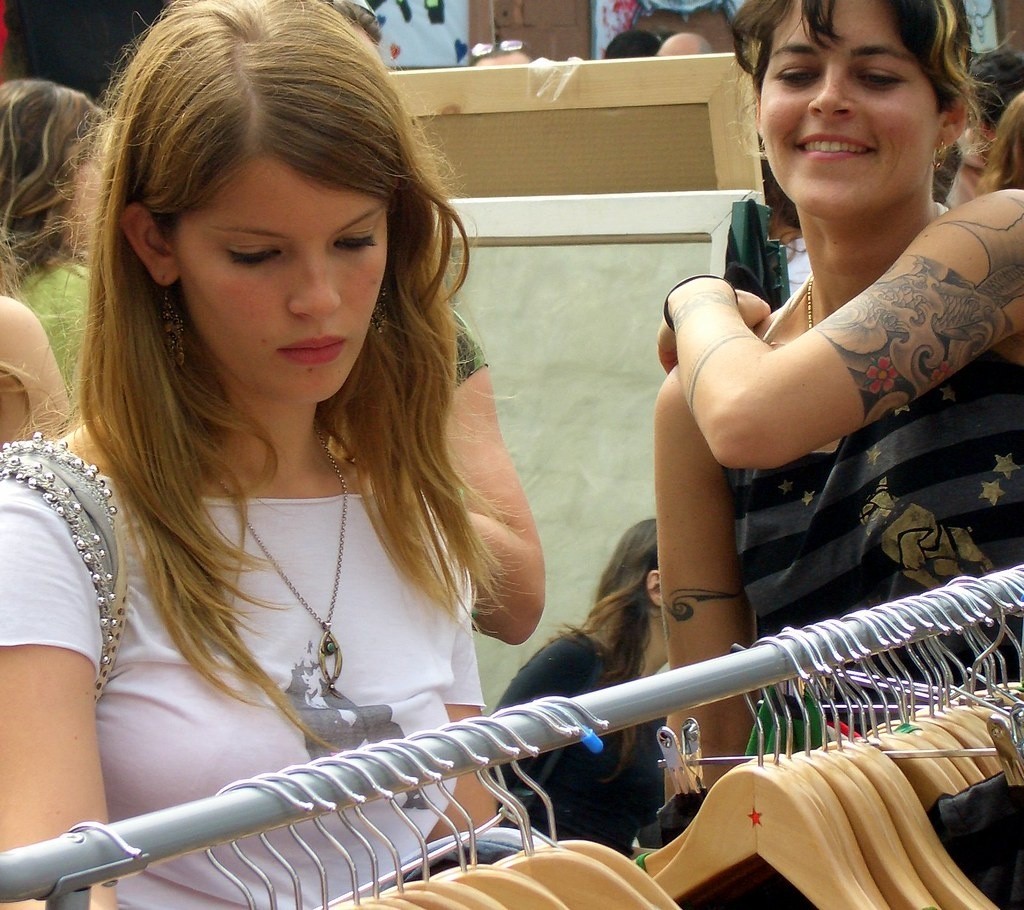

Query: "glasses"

xmin=463 ymin=40 xmax=529 ymax=65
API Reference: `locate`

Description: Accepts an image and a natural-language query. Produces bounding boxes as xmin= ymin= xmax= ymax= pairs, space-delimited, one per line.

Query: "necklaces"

xmin=807 ymin=277 xmax=816 ymax=330
xmin=218 ymin=418 xmax=348 ymax=700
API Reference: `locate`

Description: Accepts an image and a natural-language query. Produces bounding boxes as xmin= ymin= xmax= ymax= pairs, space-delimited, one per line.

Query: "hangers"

xmin=44 ymin=567 xmax=1024 ymax=910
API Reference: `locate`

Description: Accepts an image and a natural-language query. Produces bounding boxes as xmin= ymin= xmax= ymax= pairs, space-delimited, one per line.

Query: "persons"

xmin=604 ymin=27 xmax=712 ymax=59
xmin=653 ymin=1 xmax=1024 ymax=804
xmin=492 ymin=518 xmax=667 ymax=857
xmin=0 ymin=295 xmax=69 ymax=443
xmin=0 ymin=77 xmax=106 ymax=409
xmin=941 ymin=49 xmax=1024 ymax=207
xmin=467 ymin=39 xmax=536 ymax=66
xmin=444 ymin=308 xmax=546 ymax=646
xmin=0 ymin=0 xmax=498 ymax=910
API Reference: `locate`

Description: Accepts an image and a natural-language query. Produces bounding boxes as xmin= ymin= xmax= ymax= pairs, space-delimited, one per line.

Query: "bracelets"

xmin=663 ymin=274 xmax=738 ymax=332
